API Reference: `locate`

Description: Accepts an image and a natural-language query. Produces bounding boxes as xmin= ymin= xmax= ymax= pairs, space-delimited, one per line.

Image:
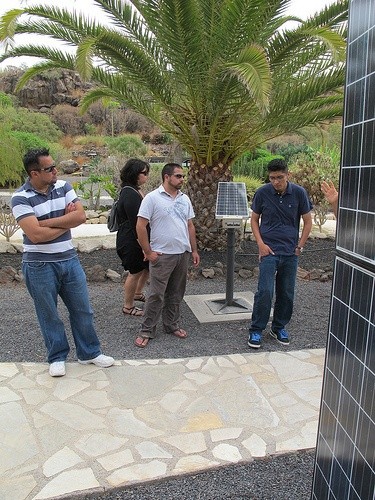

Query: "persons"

xmin=11 ymin=148 xmax=114 ymax=377
xmin=135 ymin=162 xmax=199 ymax=347
xmin=115 ymin=159 xmax=150 ymax=316
xmin=248 ymin=159 xmax=312 ymax=348
xmin=319 ymin=179 xmax=339 ymax=217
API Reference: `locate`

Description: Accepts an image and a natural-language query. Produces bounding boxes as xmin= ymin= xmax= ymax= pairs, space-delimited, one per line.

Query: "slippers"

xmin=172 ymin=328 xmax=187 ymax=338
xmin=134 ymin=335 xmax=149 ymax=348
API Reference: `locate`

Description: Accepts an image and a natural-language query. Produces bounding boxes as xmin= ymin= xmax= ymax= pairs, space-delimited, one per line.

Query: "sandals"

xmin=134 ymin=293 xmax=146 ymax=302
xmin=122 ymin=306 xmax=145 ymax=316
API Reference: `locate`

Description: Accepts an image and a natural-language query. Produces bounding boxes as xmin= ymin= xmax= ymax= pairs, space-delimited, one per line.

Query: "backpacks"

xmin=107 ymin=186 xmax=142 ymax=232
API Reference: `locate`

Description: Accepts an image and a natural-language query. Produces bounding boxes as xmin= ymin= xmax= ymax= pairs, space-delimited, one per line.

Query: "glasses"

xmin=168 ymin=174 xmax=184 ymax=179
xmin=139 ymin=171 xmax=148 ymax=176
xmin=30 ymin=163 xmax=56 ymax=173
xmin=269 ymin=175 xmax=287 ymax=181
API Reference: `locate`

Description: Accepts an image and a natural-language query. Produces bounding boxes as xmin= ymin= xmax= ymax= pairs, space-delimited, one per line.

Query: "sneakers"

xmin=270 ymin=329 xmax=289 ymax=345
xmin=78 ymin=354 xmax=115 ymax=367
xmin=248 ymin=331 xmax=262 ymax=348
xmin=49 ymin=361 xmax=65 ymax=377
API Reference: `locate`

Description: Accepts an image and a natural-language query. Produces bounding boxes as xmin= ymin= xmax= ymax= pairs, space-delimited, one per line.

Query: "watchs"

xmin=297 ymin=246 xmax=303 ymax=253
